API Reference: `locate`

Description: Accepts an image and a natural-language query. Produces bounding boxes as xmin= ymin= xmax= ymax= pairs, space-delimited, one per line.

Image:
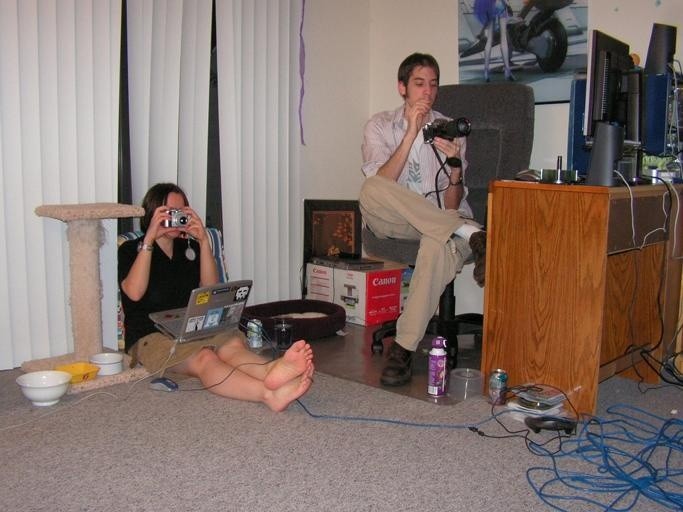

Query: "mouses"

xmin=514 ymin=169 xmax=542 ymax=183
xmin=147 ymin=377 xmax=178 ymax=392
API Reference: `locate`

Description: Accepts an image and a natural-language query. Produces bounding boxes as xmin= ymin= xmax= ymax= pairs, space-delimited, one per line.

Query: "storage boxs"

xmin=305 ymin=264 xmax=402 ymax=326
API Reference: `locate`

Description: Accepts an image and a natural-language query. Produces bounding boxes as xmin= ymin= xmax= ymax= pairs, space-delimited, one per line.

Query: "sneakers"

xmin=470 ymin=231 xmax=487 ymax=286
xmin=381 ymin=341 xmax=413 ymax=387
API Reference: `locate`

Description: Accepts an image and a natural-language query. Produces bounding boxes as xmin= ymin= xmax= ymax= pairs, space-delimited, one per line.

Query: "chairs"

xmin=362 ymin=83 xmax=534 ymax=370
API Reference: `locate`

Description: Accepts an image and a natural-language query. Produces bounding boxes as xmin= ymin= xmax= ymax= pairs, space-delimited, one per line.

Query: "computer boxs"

xmin=567 ymin=70 xmax=683 ymax=183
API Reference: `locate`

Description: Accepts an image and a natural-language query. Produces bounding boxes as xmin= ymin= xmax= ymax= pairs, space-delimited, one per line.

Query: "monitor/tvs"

xmin=583 ymin=26 xmax=648 ymax=155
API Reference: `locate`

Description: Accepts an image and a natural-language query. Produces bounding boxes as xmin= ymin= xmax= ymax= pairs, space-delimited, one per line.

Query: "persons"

xmin=358 ymin=52 xmax=487 ymax=386
xmin=118 ymin=183 xmax=315 ymax=413
xmin=473 ymin=0 xmax=517 ymax=82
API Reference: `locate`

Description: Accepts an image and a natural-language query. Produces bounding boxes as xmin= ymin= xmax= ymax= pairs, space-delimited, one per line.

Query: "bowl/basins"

xmin=15 ymin=370 xmax=73 ymax=407
xmin=87 ymin=351 xmax=123 ymax=377
xmin=450 ymin=368 xmax=485 ymax=400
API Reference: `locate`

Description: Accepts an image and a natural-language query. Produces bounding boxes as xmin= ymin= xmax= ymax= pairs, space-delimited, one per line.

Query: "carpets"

xmin=1 ymin=350 xmax=683 ymax=512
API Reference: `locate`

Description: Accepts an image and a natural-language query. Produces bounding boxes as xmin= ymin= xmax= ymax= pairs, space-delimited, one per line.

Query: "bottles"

xmin=426 ymin=334 xmax=448 ymax=398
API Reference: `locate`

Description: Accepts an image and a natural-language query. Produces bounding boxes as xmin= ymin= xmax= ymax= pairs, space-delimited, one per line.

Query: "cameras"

xmin=422 ymin=117 xmax=473 ymax=145
xmin=163 ymin=208 xmax=192 ymax=229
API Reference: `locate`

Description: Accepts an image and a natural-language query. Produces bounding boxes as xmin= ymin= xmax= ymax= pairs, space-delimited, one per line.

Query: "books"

xmin=506 ymin=382 xmax=583 ymax=424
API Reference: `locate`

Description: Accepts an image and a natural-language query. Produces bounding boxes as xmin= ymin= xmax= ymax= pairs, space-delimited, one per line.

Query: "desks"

xmin=482 ymin=180 xmax=683 ymax=423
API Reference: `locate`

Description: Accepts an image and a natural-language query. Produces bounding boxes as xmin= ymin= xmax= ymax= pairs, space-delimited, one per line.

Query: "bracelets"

xmin=137 ymin=240 xmax=154 ymax=254
xmin=450 ymin=173 xmax=463 ymax=186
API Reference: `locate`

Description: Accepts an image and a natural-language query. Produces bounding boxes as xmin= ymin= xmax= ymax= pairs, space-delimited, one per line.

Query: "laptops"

xmin=148 ymin=279 xmax=252 ymax=344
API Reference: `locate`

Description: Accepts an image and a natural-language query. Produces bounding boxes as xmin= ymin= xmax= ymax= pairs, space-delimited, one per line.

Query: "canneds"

xmin=488 ymin=370 xmax=508 ymax=405
xmin=247 ymin=319 xmax=262 ymax=348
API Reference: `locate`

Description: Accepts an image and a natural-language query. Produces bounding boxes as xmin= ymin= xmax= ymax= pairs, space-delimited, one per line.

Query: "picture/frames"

xmin=301 ymin=200 xmax=362 ymax=299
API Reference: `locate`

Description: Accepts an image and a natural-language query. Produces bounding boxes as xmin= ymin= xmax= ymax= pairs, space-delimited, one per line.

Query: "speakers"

xmin=644 ymin=22 xmax=677 ymax=76
xmin=583 ymin=119 xmax=620 ymax=185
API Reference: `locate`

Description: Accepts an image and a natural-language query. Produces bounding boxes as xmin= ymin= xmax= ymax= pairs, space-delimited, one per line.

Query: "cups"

xmin=273 ymin=317 xmax=295 ymax=356
